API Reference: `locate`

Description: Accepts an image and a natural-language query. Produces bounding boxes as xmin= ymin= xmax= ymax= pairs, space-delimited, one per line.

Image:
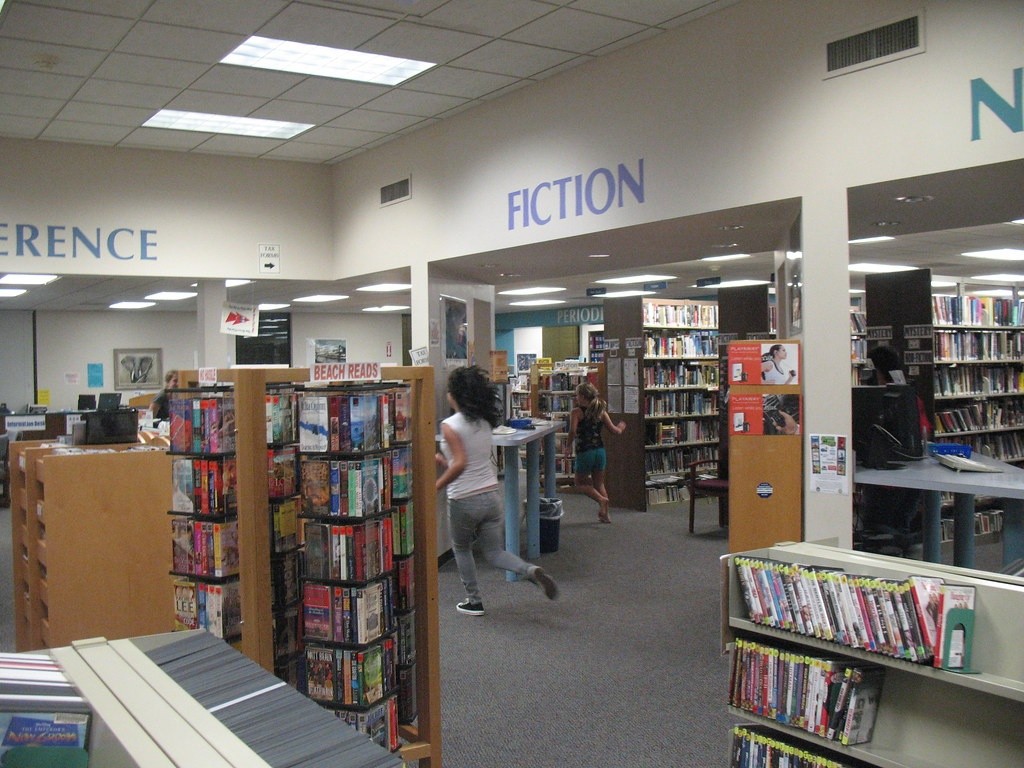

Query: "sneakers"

xmin=528 ymin=565 xmax=558 ymax=600
xmin=457 ymin=599 xmax=484 ymax=615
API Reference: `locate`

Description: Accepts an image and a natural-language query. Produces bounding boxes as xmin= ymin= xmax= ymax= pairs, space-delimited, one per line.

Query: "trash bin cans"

xmin=522 ymin=498 xmax=564 ymax=552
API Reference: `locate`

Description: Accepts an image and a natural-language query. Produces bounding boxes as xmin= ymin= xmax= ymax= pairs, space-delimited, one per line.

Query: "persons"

xmin=436 ymin=367 xmax=559 ymax=616
xmin=566 ymin=382 xmax=627 ymax=522
xmin=771 ymin=410 xmax=799 ymax=435
xmin=761 ymin=345 xmax=796 ymax=384
xmin=0 ymin=431 xmax=21 ymax=481
xmin=870 ymin=345 xmax=935 ymax=443
xmin=149 ymin=370 xmax=179 ymax=429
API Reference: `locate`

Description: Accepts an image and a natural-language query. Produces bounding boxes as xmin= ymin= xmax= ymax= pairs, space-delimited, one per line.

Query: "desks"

xmin=436 ymin=421 xmax=567 ymax=581
xmin=854 ymin=440 xmax=1024 ymax=570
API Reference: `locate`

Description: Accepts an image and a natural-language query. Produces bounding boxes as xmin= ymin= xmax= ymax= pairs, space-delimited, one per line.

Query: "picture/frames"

xmin=113 ymin=348 xmax=163 ymax=391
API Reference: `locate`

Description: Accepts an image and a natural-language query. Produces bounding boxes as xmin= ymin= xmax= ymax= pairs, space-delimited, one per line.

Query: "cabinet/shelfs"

xmin=718 ymin=289 xmax=867 ymax=528
xmin=865 ymin=268 xmax=1024 ymax=548
xmin=9 ymin=431 xmax=174 ymax=653
xmin=603 ymin=299 xmax=719 ymax=513
xmin=725 ymin=540 xmax=1024 ymax=768
xmin=165 ymin=366 xmax=441 ymax=768
xmin=511 ymin=363 xmax=603 ymax=488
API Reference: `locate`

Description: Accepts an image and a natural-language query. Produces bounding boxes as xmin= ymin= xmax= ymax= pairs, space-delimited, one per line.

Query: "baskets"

xmin=928 ymin=443 xmax=972 ymax=459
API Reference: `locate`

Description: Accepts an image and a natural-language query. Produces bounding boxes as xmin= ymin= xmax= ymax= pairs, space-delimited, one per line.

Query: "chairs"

xmin=689 ymin=460 xmax=728 ymax=534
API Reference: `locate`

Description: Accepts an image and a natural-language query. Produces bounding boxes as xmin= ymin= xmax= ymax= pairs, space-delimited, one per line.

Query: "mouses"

xmin=523 ymin=425 xmax=535 ymax=430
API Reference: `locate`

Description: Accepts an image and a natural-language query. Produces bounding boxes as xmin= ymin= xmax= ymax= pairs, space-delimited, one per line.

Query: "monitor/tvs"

xmin=98 ymin=393 xmax=122 ymax=410
xmin=78 ymin=395 xmax=96 ymax=410
xmin=851 ymin=384 xmax=924 ymax=470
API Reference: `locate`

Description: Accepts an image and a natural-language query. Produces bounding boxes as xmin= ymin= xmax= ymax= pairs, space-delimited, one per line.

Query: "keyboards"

xmin=935 ymin=453 xmax=1002 ymax=471
xmin=492 ymin=426 xmax=517 ymax=435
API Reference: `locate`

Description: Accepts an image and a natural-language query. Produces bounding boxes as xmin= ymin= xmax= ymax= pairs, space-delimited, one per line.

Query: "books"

xmin=168 ymin=396 xmax=241 ymax=655
xmin=728 ymin=554 xmax=977 ymax=768
xmin=942 ymin=492 xmax=1003 ymax=542
xmin=539 ymin=370 xmax=600 ymax=478
xmin=590 ymin=335 xmax=606 ymax=363
xmin=271 ymin=380 xmax=420 ymax=758
xmin=932 ymin=296 xmax=1024 ymax=460
xmin=849 ymin=312 xmax=868 ymax=386
xmin=643 ymin=303 xmax=721 ymax=505
xmin=144 ymin=633 xmax=406 ymax=768
xmin=0 ymin=651 xmax=92 ymax=768
xmin=510 ymin=371 xmax=533 ymax=419
